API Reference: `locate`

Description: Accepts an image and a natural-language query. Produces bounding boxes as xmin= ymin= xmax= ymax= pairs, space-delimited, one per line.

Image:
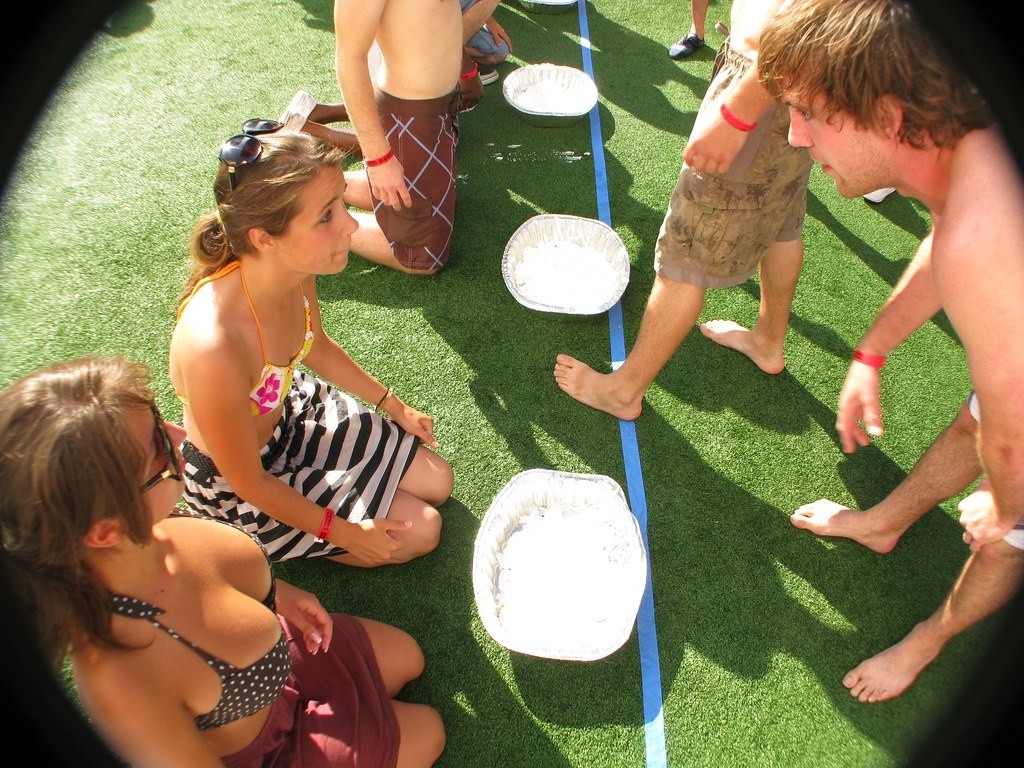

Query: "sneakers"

xmin=277 ymin=89 xmax=319 ymax=134
xmin=669 ymin=32 xmax=706 ymax=59
xmin=475 ymin=61 xmax=500 ymax=86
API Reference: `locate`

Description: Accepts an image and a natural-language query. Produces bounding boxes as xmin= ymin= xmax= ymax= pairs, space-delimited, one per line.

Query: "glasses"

xmin=97 ymin=400 xmax=183 ymax=522
xmin=217 ymin=117 xmax=285 ymax=193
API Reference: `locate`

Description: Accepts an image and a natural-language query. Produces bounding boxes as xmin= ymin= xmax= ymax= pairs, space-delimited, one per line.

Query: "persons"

xmin=759 ymin=0 xmax=1024 ymax=705
xmin=282 ymin=1 xmax=813 ymax=421
xmin=166 ymin=118 xmax=455 ymax=566
xmin=0 ymin=356 xmax=447 ymax=768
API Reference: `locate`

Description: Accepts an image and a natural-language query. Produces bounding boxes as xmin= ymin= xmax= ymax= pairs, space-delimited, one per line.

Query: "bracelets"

xmin=314 ymin=507 xmax=334 ymax=543
xmin=460 ymin=62 xmax=478 ymax=80
xmin=374 ymin=387 xmax=393 ymax=413
xmin=852 ymin=350 xmax=886 ymax=367
xmin=363 ymin=147 xmax=394 ymax=167
xmin=719 ymin=102 xmax=759 ymax=132
